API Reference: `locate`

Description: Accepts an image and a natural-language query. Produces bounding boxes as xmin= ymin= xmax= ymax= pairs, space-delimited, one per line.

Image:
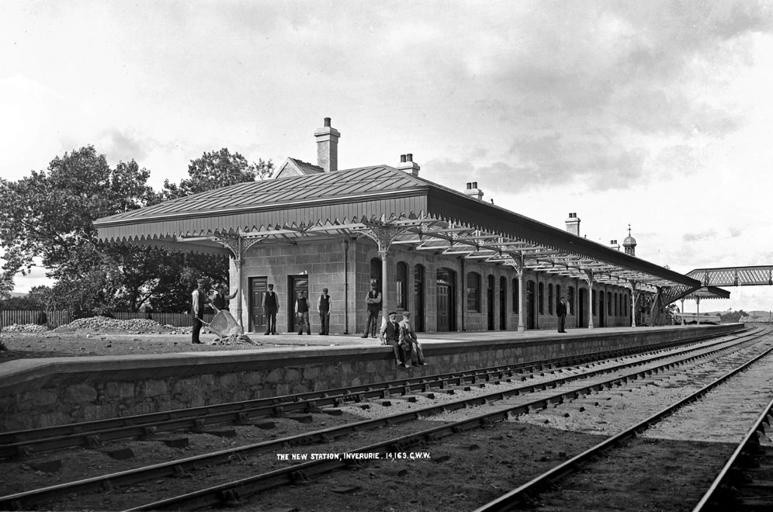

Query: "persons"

xmin=293 ymin=288 xmax=311 ymax=336
xmin=210 ymin=283 xmax=238 ymax=315
xmin=261 ymin=283 xmax=281 ymax=335
xmin=315 ymin=287 xmax=331 ymax=335
xmin=359 ymin=280 xmax=381 ymax=339
xmin=554 ymin=294 xmax=568 ymax=334
xmin=378 ymin=309 xmax=408 ymax=369
xmin=400 ymin=311 xmax=428 ymax=368
xmin=190 ymin=277 xmax=207 ymax=344
